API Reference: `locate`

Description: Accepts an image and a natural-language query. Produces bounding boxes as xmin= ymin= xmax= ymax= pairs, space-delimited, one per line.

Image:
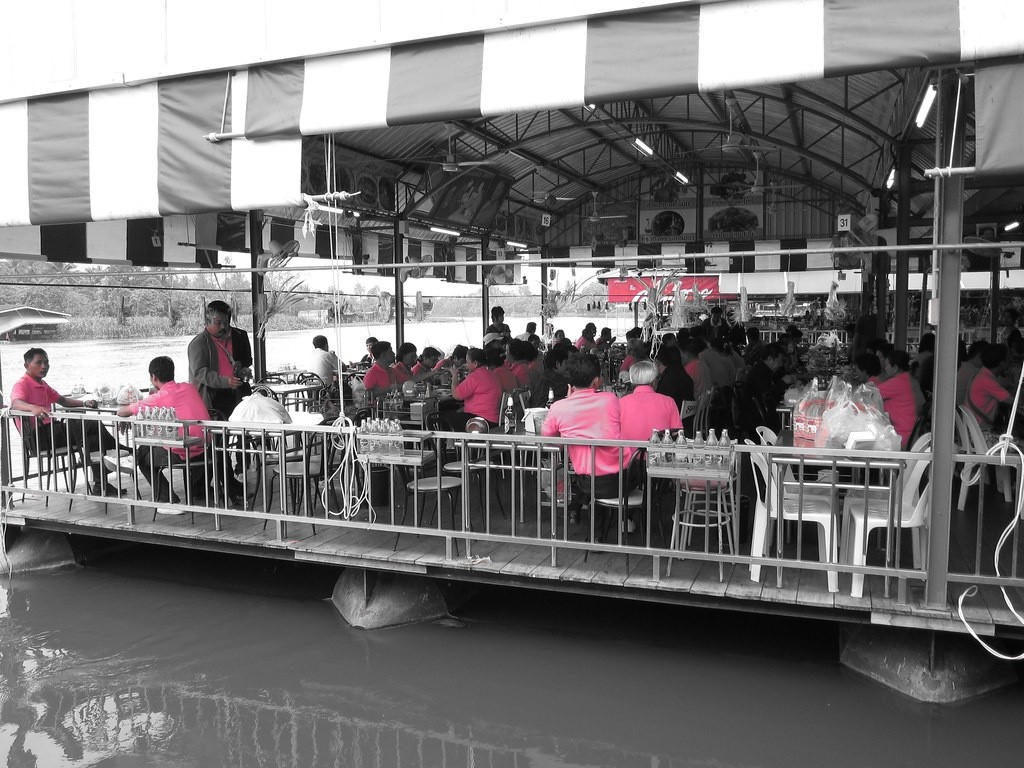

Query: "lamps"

xmin=912 ymin=84 xmax=938 ymax=129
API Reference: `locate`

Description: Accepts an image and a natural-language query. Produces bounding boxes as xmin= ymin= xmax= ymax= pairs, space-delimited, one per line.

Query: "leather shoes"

xmin=556 ymin=508 xmax=580 ymax=524
xmin=586 ymin=538 xmax=602 ymax=553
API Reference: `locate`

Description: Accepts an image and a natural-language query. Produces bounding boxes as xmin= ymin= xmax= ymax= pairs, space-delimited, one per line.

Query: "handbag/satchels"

xmin=996 ymin=416 xmax=1019 ymax=438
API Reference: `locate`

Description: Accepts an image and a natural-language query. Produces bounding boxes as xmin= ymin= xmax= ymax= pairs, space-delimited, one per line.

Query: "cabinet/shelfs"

xmin=376 ymin=396 xmax=439 ymax=451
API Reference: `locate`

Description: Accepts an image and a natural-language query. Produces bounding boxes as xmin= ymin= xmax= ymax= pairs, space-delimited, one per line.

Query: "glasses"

xmin=428 ymin=357 xmax=439 ymax=363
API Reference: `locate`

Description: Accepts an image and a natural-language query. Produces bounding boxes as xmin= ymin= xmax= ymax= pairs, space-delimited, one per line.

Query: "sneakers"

xmin=157 ymin=503 xmax=186 ymax=515
xmin=103 ymin=455 xmax=134 ymax=474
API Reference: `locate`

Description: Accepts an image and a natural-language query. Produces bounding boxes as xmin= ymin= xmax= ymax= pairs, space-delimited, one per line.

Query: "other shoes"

xmin=93 ymin=483 xmax=127 ymax=496
xmin=622 ymin=519 xmax=636 ymax=533
xmin=230 ymin=491 xmax=254 ymax=505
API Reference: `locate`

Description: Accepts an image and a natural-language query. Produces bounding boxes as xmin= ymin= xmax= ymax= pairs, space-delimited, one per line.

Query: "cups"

xmin=241 ymin=367 xmax=251 ymax=383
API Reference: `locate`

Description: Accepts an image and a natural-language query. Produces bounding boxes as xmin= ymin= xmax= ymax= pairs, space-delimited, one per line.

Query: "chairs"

xmin=9 ymin=347 xmax=1024 ymax=598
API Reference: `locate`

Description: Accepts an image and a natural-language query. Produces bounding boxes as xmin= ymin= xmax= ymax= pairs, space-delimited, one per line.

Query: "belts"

xmin=579 ymin=474 xmax=619 ymax=480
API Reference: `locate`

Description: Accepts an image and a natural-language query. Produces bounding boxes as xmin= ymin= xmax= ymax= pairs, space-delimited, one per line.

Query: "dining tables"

xmin=52 ymin=402 xmax=133 ymax=498
xmin=248 ymin=383 xmax=322 ymax=410
xmin=203 ymin=412 xmax=326 ymax=539
xmin=453 ymin=420 xmax=565 ymax=567
xmin=769 ymin=428 xmax=907 ymax=598
xmin=340 ymin=372 xmax=365 ymax=379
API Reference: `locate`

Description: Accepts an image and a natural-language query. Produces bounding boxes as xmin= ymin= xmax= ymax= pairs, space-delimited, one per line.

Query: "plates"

xmin=651 ymin=211 xmax=685 ymax=236
xmin=709 ymin=208 xmax=758 ymax=236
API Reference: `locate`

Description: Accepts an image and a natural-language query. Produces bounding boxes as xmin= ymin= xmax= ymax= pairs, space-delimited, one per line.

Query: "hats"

xmin=483 ymin=333 xmax=503 ymax=345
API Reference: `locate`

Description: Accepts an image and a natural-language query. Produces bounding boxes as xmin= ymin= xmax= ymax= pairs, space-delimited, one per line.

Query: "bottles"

xmin=692 ymin=431 xmax=706 ymax=470
xmin=136 ymin=406 xmax=178 ymax=437
xmin=505 ymin=395 xmax=518 ymax=436
xmin=648 ymin=429 xmax=661 ymax=467
xmin=612 ymin=380 xmax=618 ymax=396
xmin=545 ymin=388 xmax=557 ymax=409
xmin=277 ymin=362 xmax=297 ymax=381
xmin=675 ymin=430 xmax=688 ymax=469
xmin=707 ymin=429 xmax=717 ymax=469
xmin=662 ymin=429 xmax=673 ymax=469
xmin=383 ymin=391 xmax=403 ymax=411
xmin=357 ymin=418 xmax=404 ymax=456
xmin=718 ymin=430 xmax=732 ymax=469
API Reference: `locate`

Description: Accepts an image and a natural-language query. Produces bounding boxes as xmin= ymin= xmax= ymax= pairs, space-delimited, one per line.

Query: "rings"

xmin=41 ymin=412 xmax=46 ymax=417
xmin=39 ymin=416 xmax=43 ymax=419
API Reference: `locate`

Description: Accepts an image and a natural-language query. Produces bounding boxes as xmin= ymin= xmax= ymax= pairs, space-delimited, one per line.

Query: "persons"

xmin=9 ymin=347 xmax=132 ymax=497
xmin=112 ymin=356 xmax=214 ymax=515
xmin=440 ymin=179 xmax=508 ymax=232
xmin=186 ymin=299 xmax=1024 ymax=537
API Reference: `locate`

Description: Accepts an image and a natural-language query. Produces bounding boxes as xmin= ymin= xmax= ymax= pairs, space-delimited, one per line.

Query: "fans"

xmin=399 ymin=255 xmax=432 ymax=283
xmin=963 ymin=227 xmax=1005 ymax=258
xmin=828 ymin=213 xmax=880 ymax=272
xmin=399 ymin=97 xmax=809 ymax=223
xmin=482 ymin=264 xmax=514 ymax=288
xmin=257 ymin=238 xmax=300 ymax=277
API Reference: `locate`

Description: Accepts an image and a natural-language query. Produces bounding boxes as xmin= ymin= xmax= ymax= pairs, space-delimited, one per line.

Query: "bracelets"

xmin=82 ymin=400 xmax=86 ymax=406
